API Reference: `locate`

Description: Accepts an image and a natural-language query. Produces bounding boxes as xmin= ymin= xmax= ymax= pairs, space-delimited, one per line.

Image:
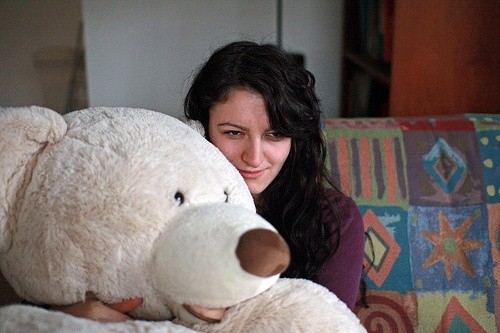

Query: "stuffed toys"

xmin=1 ymin=106 xmax=367 ymax=333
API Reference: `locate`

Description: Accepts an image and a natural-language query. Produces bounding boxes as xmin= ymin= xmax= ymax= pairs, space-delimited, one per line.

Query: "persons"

xmin=50 ymin=40 xmax=365 ymax=322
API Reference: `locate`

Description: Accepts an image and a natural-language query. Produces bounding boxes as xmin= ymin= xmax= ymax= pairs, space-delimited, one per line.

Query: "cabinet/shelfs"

xmin=337 ymin=0 xmax=500 ymax=121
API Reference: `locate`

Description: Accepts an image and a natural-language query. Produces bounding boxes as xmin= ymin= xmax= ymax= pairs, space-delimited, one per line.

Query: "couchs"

xmin=308 ymin=113 xmax=500 ymax=333
